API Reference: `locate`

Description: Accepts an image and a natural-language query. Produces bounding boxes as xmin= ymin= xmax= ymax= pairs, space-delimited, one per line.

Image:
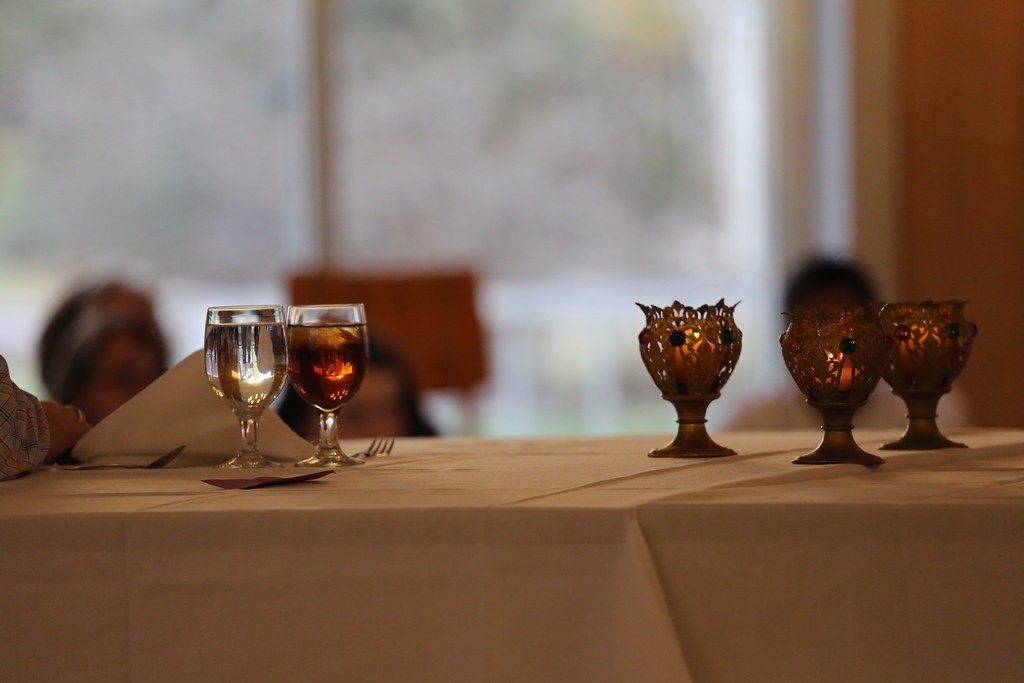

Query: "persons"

xmin=729 ymin=256 xmax=904 ymax=436
xmin=1 ymin=287 xmax=441 ymax=481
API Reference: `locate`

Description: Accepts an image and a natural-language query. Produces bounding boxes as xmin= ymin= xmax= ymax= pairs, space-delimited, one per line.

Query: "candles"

xmin=813 ymin=350 xmax=863 ymax=391
xmin=673 ymin=326 xmax=714 ymax=373
xmin=894 ymin=319 xmax=940 ymax=370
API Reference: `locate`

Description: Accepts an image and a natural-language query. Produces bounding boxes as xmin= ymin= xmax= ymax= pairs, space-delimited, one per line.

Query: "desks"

xmin=0 ymin=430 xmax=1024 ymax=683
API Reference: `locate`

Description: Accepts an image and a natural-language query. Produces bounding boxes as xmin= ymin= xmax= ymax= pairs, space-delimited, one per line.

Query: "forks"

xmin=347 ymin=435 xmax=396 ymax=459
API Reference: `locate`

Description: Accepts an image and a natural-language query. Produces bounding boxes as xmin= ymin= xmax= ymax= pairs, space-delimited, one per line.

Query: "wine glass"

xmin=285 ymin=304 xmax=370 ymax=467
xmin=204 ymin=304 xmax=289 ymax=468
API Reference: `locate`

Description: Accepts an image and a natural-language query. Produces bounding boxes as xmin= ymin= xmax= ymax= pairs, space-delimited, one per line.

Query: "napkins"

xmin=71 ymin=348 xmax=317 ymax=465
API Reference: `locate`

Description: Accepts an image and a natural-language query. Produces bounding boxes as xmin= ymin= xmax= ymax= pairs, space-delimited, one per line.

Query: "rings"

xmin=66 ymin=405 xmax=86 ymax=423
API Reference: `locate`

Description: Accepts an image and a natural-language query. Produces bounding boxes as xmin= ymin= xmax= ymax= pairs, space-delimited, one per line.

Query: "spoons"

xmin=37 ymin=443 xmax=187 ymax=471
